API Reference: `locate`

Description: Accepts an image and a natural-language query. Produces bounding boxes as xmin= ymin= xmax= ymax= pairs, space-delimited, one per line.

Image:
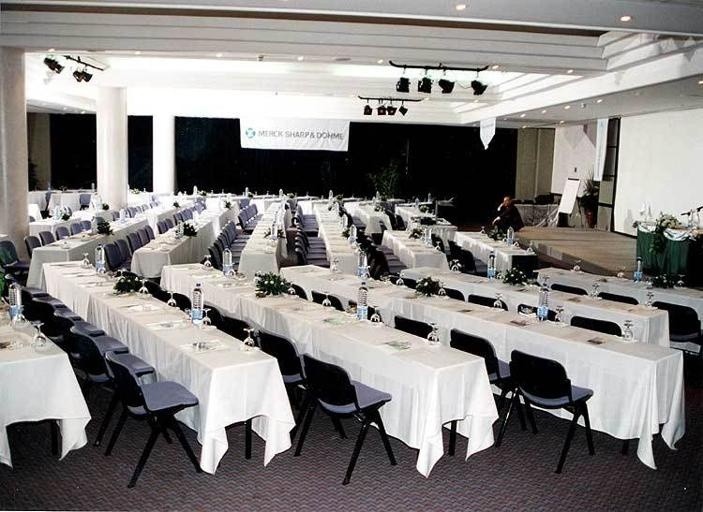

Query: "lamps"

xmin=358 ymin=96 xmax=421 ymax=116
xmin=389 ymin=60 xmax=489 ymax=96
xmin=44 ymin=55 xmax=104 ymax=82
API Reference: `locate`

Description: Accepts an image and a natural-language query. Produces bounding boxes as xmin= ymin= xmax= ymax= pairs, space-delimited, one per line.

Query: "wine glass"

xmin=0 ymin=181 xmax=689 ymax=358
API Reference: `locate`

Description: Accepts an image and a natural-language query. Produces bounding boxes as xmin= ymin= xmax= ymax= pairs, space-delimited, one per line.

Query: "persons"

xmin=492 ymin=196 xmax=524 ymax=234
